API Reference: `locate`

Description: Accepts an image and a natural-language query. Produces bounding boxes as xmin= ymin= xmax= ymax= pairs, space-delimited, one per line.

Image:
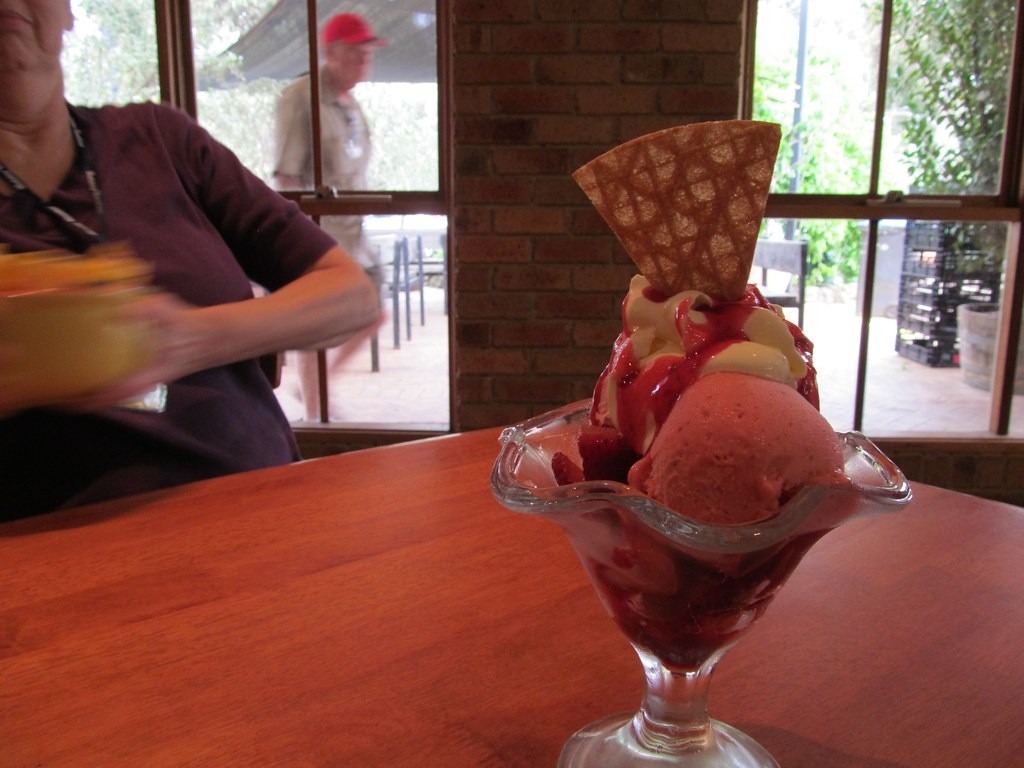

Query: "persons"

xmin=272 ymin=14 xmax=388 ymax=403
xmin=0 ymin=0 xmax=382 ymax=521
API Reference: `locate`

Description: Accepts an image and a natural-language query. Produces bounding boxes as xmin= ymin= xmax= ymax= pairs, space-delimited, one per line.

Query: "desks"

xmin=0 ymin=418 xmax=1024 ymax=767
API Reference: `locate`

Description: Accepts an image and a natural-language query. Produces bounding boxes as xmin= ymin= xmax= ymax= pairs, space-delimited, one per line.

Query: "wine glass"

xmin=492 ymin=400 xmax=911 ymax=768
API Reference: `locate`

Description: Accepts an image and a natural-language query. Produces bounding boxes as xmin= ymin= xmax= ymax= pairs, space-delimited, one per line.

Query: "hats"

xmin=320 ymin=12 xmax=387 ymax=45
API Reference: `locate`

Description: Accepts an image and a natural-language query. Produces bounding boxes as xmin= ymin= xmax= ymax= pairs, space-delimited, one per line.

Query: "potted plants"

xmin=954 ymin=161 xmax=1023 ymax=397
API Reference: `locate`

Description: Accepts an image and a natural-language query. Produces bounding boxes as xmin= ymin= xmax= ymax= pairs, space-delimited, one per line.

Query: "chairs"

xmin=366 ymin=230 xmax=447 ymax=372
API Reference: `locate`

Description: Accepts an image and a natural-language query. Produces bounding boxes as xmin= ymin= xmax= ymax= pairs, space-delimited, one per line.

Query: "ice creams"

xmin=551 ymin=118 xmax=847 ymax=676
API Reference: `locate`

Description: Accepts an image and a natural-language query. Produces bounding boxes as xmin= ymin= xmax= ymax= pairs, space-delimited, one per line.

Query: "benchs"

xmin=750 ymin=241 xmax=810 ymax=330
